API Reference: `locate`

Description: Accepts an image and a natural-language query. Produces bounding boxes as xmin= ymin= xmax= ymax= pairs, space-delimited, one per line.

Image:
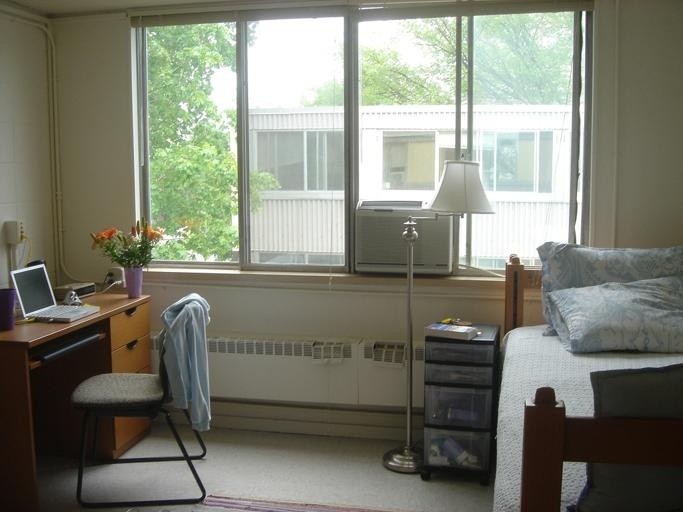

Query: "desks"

xmin=0 ymin=290 xmax=151 ymax=512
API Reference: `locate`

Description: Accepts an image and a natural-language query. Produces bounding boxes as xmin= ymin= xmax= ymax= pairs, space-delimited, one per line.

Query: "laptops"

xmin=10 ymin=263 xmax=101 ymax=323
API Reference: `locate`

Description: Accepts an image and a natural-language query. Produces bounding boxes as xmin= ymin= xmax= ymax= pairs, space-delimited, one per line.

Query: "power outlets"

xmin=3 ymin=221 xmax=23 ymax=246
xmin=109 ymin=267 xmax=127 ymax=289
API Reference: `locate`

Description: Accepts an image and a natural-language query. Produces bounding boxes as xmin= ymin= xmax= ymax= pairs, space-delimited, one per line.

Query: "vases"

xmin=124 ymin=267 xmax=143 ymax=298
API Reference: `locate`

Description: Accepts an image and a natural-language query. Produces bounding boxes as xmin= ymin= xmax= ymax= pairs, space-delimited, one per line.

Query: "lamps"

xmin=383 ymin=153 xmax=495 ymax=474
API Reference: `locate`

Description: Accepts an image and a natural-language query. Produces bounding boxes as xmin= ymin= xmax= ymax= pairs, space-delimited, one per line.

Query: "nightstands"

xmin=421 ymin=324 xmax=500 ymax=485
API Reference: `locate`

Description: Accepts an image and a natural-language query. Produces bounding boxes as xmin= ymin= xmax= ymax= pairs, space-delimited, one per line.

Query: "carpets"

xmin=192 ymin=495 xmax=392 ymax=512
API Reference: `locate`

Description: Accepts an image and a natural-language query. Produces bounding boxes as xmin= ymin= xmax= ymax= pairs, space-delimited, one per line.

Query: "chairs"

xmin=71 ymin=293 xmax=211 ymax=508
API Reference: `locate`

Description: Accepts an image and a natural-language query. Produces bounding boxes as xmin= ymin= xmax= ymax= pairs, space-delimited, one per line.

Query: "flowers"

xmin=90 ymin=216 xmax=166 ymax=272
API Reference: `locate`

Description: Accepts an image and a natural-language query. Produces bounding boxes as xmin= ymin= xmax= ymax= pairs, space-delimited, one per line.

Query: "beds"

xmin=492 ymin=253 xmax=683 ymax=512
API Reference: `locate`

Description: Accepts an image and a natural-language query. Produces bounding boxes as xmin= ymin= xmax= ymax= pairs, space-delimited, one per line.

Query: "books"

xmin=425 ymin=322 xmax=478 ymax=341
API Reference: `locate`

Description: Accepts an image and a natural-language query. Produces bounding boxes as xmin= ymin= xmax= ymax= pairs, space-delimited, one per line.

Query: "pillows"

xmin=545 ymin=277 xmax=683 ymax=354
xmin=537 ymin=242 xmax=683 ymax=335
xmin=566 ymin=363 xmax=683 ymax=512
